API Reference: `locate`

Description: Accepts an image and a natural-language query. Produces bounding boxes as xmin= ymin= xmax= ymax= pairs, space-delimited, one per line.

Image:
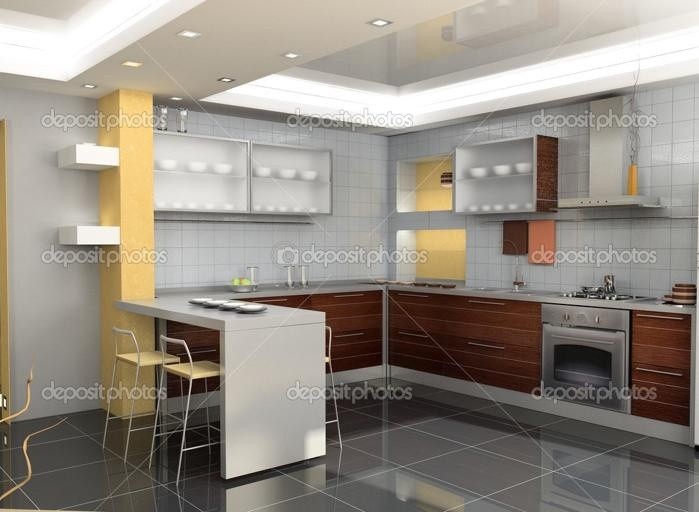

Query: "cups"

xmin=248 ymin=264 xmax=308 ymax=287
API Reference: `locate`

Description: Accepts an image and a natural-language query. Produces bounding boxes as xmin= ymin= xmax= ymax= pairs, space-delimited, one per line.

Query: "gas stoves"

xmin=563 ymin=287 xmax=657 ymax=303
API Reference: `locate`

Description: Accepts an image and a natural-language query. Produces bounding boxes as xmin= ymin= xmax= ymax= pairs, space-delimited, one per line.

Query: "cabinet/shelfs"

xmin=57 ymin=142 xmax=121 ymax=245
xmin=628 ymin=310 xmax=691 ymax=447
xmin=166 ymin=289 xmax=382 ymax=414
xmin=152 ymin=127 xmax=336 ymax=218
xmin=451 ymin=133 xmax=557 ymax=216
xmin=388 ymin=290 xmax=541 ymax=413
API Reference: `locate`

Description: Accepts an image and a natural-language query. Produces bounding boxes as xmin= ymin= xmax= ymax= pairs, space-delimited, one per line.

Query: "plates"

xmin=190 ymin=298 xmax=264 ymax=312
xmin=375 ymin=280 xmax=456 ymax=288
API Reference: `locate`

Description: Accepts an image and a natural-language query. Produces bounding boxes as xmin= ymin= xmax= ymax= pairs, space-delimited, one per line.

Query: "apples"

xmin=232 ymin=277 xmax=240 ymax=285
xmin=241 ymin=278 xmax=251 ymax=285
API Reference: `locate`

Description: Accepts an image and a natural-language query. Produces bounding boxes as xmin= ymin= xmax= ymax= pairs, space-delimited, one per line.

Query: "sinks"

xmin=472 ymin=286 xmax=523 ymax=291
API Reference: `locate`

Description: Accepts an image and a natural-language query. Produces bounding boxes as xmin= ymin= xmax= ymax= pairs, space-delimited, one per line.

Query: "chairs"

xmin=101 ymin=326 xmax=221 ymax=486
xmin=325 ymin=325 xmax=343 ymax=450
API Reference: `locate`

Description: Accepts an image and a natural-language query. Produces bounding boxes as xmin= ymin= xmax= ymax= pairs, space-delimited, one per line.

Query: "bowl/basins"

xmin=154 ymin=158 xmax=318 ymax=182
xmin=469 ymin=160 xmax=532 ymax=211
xmin=672 ymin=287 xmax=696 ymax=305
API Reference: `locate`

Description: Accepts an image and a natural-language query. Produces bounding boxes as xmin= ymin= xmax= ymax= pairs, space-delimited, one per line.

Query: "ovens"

xmin=542 ymin=322 xmax=627 ymax=411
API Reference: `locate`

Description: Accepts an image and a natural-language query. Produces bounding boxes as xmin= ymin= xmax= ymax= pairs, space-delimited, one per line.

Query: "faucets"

xmin=501 ymin=239 xmax=527 ymax=290
